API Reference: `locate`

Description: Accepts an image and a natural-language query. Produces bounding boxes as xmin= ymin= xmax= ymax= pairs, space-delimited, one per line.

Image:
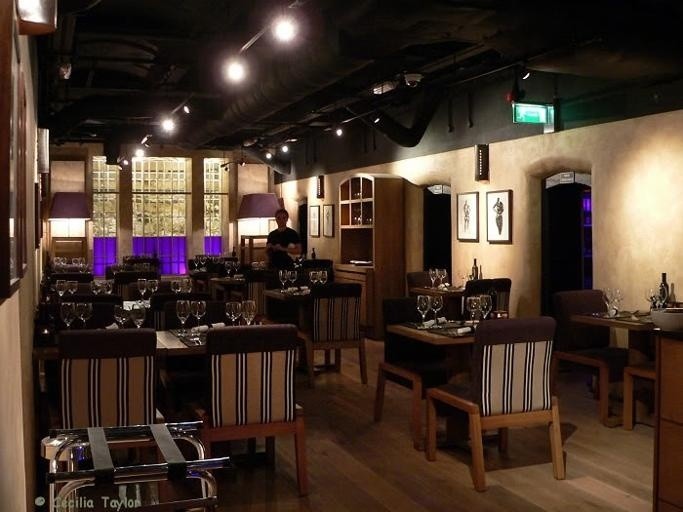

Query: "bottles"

xmin=311 ymin=247 xmax=316 ymax=259
xmin=232 ymin=247 xmax=236 ymax=257
xmin=471 ymin=258 xmax=478 ymax=279
xmin=660 ymin=273 xmax=669 ymax=304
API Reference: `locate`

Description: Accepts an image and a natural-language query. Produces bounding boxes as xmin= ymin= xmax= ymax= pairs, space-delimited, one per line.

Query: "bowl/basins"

xmin=650 ymin=308 xmax=683 ymax=332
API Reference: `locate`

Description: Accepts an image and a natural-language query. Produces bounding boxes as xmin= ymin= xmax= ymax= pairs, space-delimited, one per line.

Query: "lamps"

xmin=375 ymin=114 xmax=416 ymax=147
xmin=474 ymin=144 xmax=489 ymax=180
xmin=512 ymin=64 xmax=532 ymax=79
xmin=223 ymin=152 xmax=229 ymax=171
xmin=237 ymin=160 xmax=245 ymax=167
xmin=46 ymin=192 xmax=94 ymax=267
xmin=236 ymin=193 xmax=281 ymax=264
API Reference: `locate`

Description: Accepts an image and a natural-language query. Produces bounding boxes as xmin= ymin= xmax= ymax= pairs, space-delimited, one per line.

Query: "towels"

xmin=288 ymin=286 xmax=308 ymax=290
xmin=287 ymin=244 xmax=301 ymax=261
xmin=293 ymin=289 xmax=310 ymax=296
xmin=422 ymin=317 xmax=446 ymax=328
xmin=191 ymin=322 xmax=225 ymax=332
xmin=610 ymin=306 xmax=618 ymax=317
xmin=458 ymin=325 xmax=476 ymax=334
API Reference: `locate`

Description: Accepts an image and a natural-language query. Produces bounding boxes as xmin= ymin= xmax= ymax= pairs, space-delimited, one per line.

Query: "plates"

xmin=349 ymin=260 xmax=372 ymax=265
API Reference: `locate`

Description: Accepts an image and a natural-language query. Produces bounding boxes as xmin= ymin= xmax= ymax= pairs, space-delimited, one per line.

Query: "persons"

xmin=265 ymin=209 xmax=303 ymax=282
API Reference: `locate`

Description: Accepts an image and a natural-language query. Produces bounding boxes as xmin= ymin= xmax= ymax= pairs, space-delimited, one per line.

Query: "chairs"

xmin=622 ymin=368 xmax=655 ymax=429
xmin=165 ymin=302 xmax=239 ymax=329
xmin=298 ymin=283 xmax=368 ymax=388
xmin=129 ymin=282 xmax=171 ymax=300
xmin=407 ymin=270 xmax=449 ymax=316
xmin=246 ymin=270 xmax=271 ymax=316
xmin=428 ymin=318 xmax=564 ymax=492
xmin=152 ymin=294 xmax=211 ymax=327
xmin=302 ymin=258 xmax=335 ymax=286
xmin=114 ymin=272 xmax=160 ymax=300
xmin=62 ymin=329 xmax=156 ymax=426
xmin=556 ymin=290 xmax=631 ymax=427
xmin=36 ymin=303 xmax=154 ymax=347
xmin=190 ymin=325 xmax=309 ymax=496
xmin=374 ymin=299 xmax=422 ymax=450
xmin=465 ymin=278 xmax=511 ymax=316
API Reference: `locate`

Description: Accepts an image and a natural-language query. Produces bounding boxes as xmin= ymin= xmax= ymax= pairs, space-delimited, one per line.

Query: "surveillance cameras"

xmin=403 ymin=74 xmax=422 ymax=88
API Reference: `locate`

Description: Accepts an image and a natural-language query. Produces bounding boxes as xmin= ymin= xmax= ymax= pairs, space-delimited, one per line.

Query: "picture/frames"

xmin=323 ymin=204 xmax=334 ymax=237
xmin=485 ymin=189 xmax=512 ymax=244
xmin=309 ymin=206 xmax=319 ymax=237
xmin=35 ymin=182 xmax=41 ymax=249
xmin=0 ymin=1 xmax=28 ymax=299
xmin=456 ymin=191 xmax=479 ymax=242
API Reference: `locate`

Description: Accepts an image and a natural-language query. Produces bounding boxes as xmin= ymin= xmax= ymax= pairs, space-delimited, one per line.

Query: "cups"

xmin=613 ymin=287 xmax=625 ymax=317
xmin=192 ymin=255 xmax=199 ymax=271
xmin=428 ymin=294 xmax=443 ymax=329
xmin=438 ymin=269 xmax=446 ymax=284
xmin=113 ymin=303 xmax=130 ymax=329
xmin=416 ymin=295 xmax=428 ymax=328
xmin=199 ymin=255 xmax=205 ymax=266
xmin=652 ymin=281 xmax=667 ymax=309
xmin=175 ymin=300 xmax=190 ymax=337
xmin=146 ymin=280 xmax=158 ymax=293
xmin=601 ymin=287 xmax=616 ymax=318
xmin=71 ymin=258 xmax=78 ymax=267
xmin=318 ymin=270 xmax=327 ymax=284
xmin=55 ymin=280 xmax=66 ymax=297
xmin=60 ymin=302 xmax=75 ymax=330
xmin=170 ymin=280 xmax=181 ymax=293
xmin=101 ymin=280 xmax=114 ymax=295
xmin=60 ymin=257 xmax=67 ymax=266
xmin=232 ymin=261 xmax=240 ymax=271
xmin=644 ymin=287 xmax=658 ymax=309
xmin=90 ymin=280 xmax=102 ymax=295
xmin=224 ymin=261 xmax=232 ymax=275
xmin=224 ymin=302 xmax=241 ymax=326
xmin=78 ymin=257 xmax=85 ymax=267
xmin=52 ymin=257 xmax=60 ymax=266
xmin=240 ymin=300 xmax=257 ymax=325
xmin=131 ymin=303 xmax=146 ymax=328
xmin=75 ymin=302 xmax=92 ymax=329
xmin=428 ymin=269 xmax=438 ymax=290
xmin=190 ymin=300 xmax=206 ymax=336
xmin=288 ymin=271 xmax=297 ymax=287
xmin=466 ymin=296 xmax=480 ymax=331
xmin=278 ymin=270 xmax=288 ymax=292
xmin=309 ymin=272 xmax=318 ymax=284
xmin=66 ymin=281 xmax=78 ymax=294
xmin=480 ymin=295 xmax=492 ymax=319
xmin=181 ymin=279 xmax=192 ymax=293
xmin=137 ymin=279 xmax=146 ymax=304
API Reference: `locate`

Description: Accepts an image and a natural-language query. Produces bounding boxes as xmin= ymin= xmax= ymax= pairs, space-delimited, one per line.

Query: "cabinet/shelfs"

xmin=333 ymin=172 xmax=423 ymax=339
xmin=653 ymin=328 xmax=682 ymax=512
